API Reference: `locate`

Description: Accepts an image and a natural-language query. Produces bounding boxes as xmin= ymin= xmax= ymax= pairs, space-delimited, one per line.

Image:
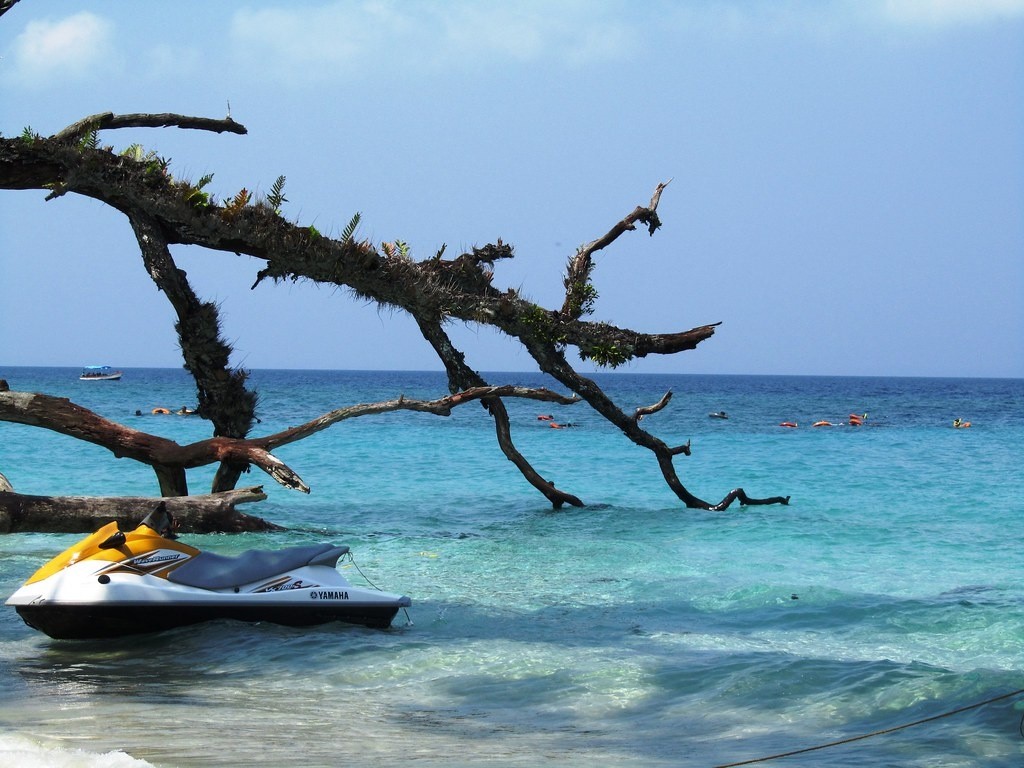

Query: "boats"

xmin=79 ymin=366 xmax=122 ymax=381
xmin=4 ymin=499 xmax=414 ymax=643
